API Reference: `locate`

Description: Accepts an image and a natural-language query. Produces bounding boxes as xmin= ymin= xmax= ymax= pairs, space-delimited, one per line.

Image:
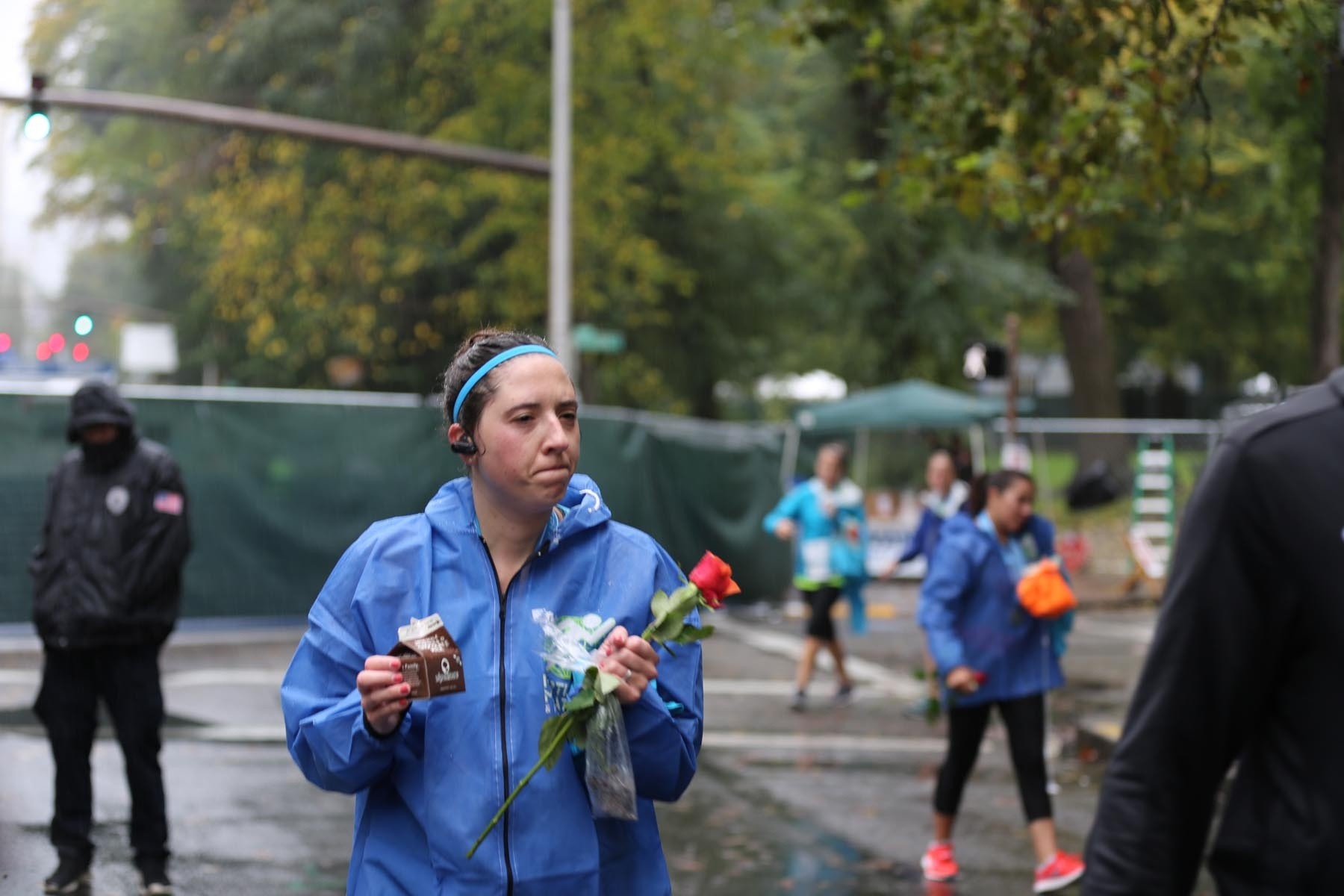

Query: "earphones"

xmin=450 ymin=434 xmax=477 ymax=455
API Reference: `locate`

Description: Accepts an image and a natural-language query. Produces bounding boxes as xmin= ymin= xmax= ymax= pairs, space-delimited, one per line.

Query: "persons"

xmin=884 ymin=451 xmax=968 ymax=726
xmin=764 ymin=439 xmax=866 ymax=714
xmin=1076 ymin=367 xmax=1344 ymax=896
xmin=31 ymin=386 xmax=195 ymax=896
xmin=920 ymin=468 xmax=1082 ymax=891
xmin=281 ymin=327 xmax=702 ymax=896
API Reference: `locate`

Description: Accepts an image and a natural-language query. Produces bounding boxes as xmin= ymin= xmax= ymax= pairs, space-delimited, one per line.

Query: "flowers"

xmin=466 ymin=551 xmax=741 ymax=861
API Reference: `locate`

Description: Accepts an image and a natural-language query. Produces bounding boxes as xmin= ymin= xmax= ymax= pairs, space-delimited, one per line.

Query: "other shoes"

xmin=921 ymin=846 xmax=959 ymax=882
xmin=1032 ymin=849 xmax=1086 ymax=894
xmin=134 ymin=850 xmax=174 ymax=895
xmin=44 ymin=847 xmax=93 ymax=894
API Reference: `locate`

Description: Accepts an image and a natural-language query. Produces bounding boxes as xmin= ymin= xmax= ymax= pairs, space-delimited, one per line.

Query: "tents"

xmin=794 ymin=381 xmax=1001 ymax=478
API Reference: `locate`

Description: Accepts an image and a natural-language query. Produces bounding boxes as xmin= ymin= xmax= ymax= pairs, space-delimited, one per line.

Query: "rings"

xmin=621 ymin=669 xmax=634 ymax=683
